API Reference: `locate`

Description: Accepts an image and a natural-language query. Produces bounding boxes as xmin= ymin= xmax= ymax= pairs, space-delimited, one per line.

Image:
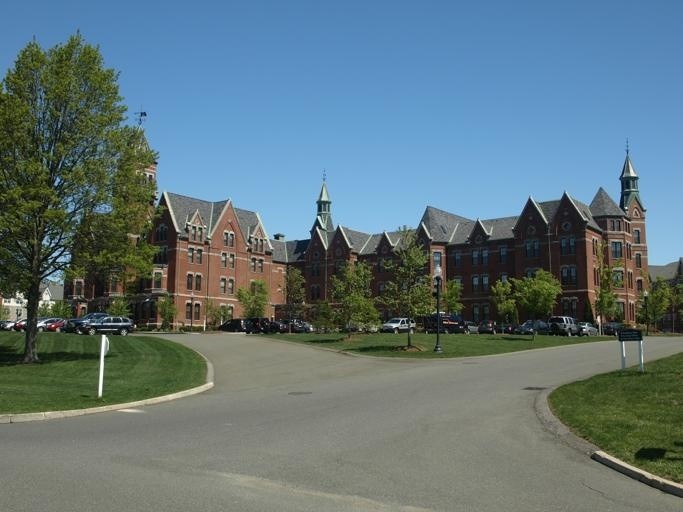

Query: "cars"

xmin=0 ymin=312 xmax=137 ymax=336
xmin=217 ymin=317 xmax=317 ymax=333
xmin=382 ymin=317 xmax=416 ymax=334
xmin=467 ymin=315 xmax=598 ymax=336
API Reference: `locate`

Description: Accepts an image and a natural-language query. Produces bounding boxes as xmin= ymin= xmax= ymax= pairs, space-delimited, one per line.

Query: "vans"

xmin=424 ymin=312 xmax=470 ymax=334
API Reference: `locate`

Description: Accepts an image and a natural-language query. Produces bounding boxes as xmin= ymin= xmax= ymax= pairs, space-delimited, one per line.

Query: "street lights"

xmin=432 ymin=263 xmax=444 ymax=351
